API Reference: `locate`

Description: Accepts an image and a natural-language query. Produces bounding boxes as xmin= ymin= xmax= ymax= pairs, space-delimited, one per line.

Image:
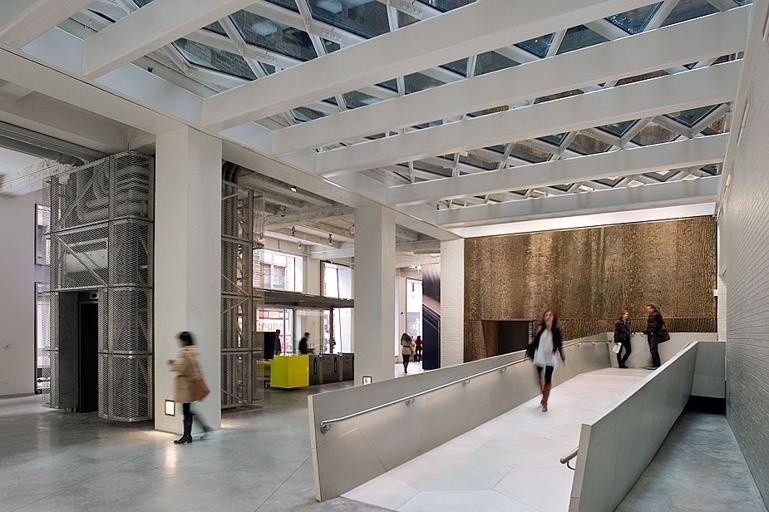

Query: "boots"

xmin=174 ymin=414 xmax=213 ymax=444
xmin=539 ymin=384 xmax=551 ymax=412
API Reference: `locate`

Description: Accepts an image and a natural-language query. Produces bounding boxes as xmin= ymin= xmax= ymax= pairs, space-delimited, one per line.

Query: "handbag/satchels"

xmin=192 ymin=378 xmax=210 ymax=401
xmin=526 ymin=337 xmax=536 ymax=359
xmin=657 ymin=329 xmax=670 ymax=343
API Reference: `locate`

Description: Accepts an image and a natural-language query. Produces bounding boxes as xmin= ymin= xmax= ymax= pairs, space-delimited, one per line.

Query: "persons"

xmin=526 ymin=309 xmax=566 ymax=412
xmin=400 ymin=333 xmax=415 ymax=373
xmin=167 ymin=331 xmax=214 ymax=444
xmin=614 ymin=311 xmax=632 ymax=368
xmin=415 ymin=336 xmax=423 ymax=362
xmin=299 ymin=332 xmax=311 ymax=354
xmin=274 ymin=329 xmax=281 ymax=355
xmin=640 ymin=303 xmax=663 ymax=369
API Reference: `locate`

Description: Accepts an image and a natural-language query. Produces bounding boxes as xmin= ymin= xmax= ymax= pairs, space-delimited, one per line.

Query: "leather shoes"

xmin=647 ymin=365 xmax=659 ymax=369
xmin=619 ymin=364 xmax=628 ymax=368
